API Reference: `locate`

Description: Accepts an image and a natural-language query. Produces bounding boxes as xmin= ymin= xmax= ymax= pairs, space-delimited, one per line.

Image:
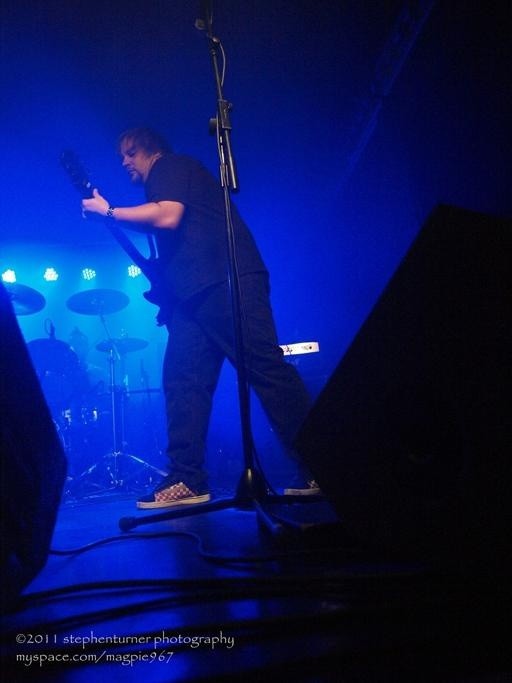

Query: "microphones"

xmin=195 ymin=1 xmax=213 ymax=34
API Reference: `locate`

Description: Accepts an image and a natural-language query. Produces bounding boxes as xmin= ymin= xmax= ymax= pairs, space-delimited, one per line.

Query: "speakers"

xmin=295 ymin=200 xmax=510 ymax=623
xmin=1 ymin=282 xmax=67 ymax=601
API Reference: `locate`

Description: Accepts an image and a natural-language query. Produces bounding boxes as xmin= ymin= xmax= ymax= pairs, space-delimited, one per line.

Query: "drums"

xmin=28 ymin=338 xmax=78 ymax=425
xmin=78 ymin=405 xmax=100 ymax=425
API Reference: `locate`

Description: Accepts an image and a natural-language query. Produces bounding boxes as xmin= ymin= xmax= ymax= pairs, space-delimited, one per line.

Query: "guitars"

xmin=47 ymin=149 xmax=176 ymax=333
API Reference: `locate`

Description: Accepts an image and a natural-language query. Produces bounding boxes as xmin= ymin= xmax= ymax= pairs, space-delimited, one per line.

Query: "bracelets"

xmin=107 ymin=207 xmax=114 ymax=216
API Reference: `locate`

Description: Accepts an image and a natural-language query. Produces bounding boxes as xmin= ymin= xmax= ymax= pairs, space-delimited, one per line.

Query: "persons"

xmin=80 ymin=122 xmax=321 ymax=510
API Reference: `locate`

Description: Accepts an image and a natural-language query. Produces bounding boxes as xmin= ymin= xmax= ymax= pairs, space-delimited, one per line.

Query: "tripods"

xmin=68 ymin=345 xmax=169 ymax=489
xmin=120 ymin=114 xmax=327 ymax=550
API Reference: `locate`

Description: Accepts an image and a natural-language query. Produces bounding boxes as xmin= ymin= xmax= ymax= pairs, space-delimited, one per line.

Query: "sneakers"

xmin=136 ymin=476 xmax=211 ymax=509
xmin=285 ymin=479 xmax=319 ymax=497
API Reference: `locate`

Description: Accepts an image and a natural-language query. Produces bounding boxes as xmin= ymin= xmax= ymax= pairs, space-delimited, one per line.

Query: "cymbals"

xmin=67 ymin=289 xmax=129 ymax=315
xmin=96 ymin=338 xmax=148 ymax=353
xmin=4 ymin=286 xmax=46 ymax=314
